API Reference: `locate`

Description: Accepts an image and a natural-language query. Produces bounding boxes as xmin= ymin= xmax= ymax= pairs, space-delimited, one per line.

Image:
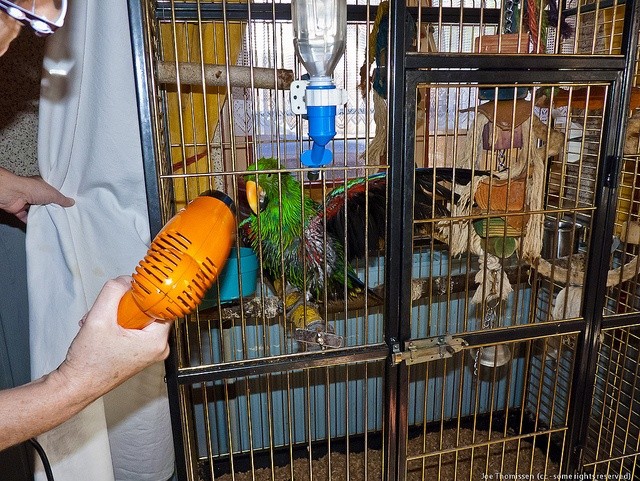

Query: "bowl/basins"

xmin=207 ymin=246 xmax=258 ymax=300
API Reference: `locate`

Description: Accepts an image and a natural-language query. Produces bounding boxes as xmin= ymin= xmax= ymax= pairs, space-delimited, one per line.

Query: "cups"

xmin=542 ymin=223 xmax=584 ymax=258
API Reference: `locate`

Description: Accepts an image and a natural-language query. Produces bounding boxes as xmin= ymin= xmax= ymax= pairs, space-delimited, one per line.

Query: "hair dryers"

xmin=116 ymin=188 xmax=239 ymax=329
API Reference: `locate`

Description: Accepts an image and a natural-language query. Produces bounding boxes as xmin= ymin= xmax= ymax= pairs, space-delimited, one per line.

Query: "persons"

xmin=0 ymin=165 xmax=173 ymax=453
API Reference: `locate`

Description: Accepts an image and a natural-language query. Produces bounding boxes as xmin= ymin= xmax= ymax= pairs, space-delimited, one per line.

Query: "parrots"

xmin=237 ymin=155 xmax=500 ymax=320
